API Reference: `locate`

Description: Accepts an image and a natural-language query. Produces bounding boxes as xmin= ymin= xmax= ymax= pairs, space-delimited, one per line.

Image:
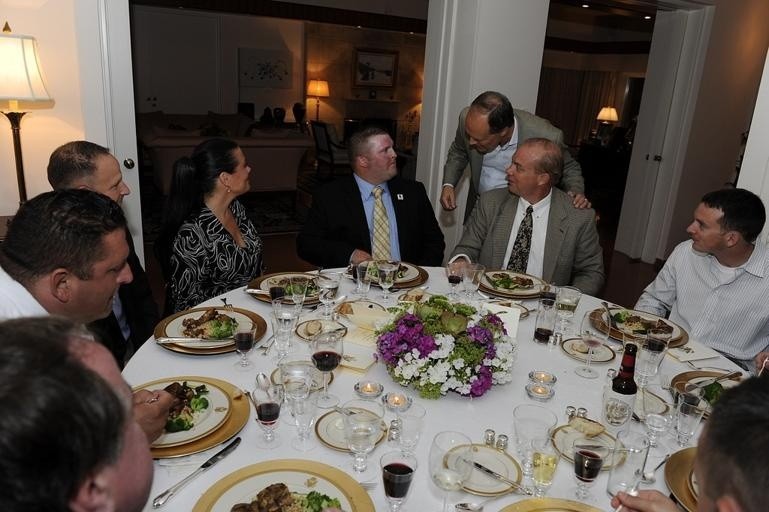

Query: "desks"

xmin=137 ymin=110 xmax=319 ymax=227
xmin=0 ymin=216 xmax=17 ymax=242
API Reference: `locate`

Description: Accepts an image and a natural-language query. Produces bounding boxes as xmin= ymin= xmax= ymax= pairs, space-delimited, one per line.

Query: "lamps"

xmin=596 ymin=105 xmax=619 ymax=127
xmin=0 ymin=21 xmax=56 ymax=209
xmin=304 ymin=78 xmax=330 ymax=122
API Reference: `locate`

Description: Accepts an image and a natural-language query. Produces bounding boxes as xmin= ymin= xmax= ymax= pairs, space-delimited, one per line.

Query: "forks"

xmin=660 ymin=375 xmax=676 ymax=400
xmin=220 ymin=298 xmax=233 ymax=312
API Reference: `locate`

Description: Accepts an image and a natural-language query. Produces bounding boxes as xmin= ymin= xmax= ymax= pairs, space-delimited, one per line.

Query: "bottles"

xmin=532 ymin=298 xmax=559 ymax=346
xmin=600 ymin=343 xmax=638 ymax=439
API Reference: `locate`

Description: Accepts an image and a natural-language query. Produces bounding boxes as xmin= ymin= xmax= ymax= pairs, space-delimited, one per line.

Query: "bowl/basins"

xmin=353 ymin=380 xmax=384 ymax=399
xmin=381 ymin=391 xmax=413 ymax=413
xmin=524 ymin=370 xmax=558 ymax=403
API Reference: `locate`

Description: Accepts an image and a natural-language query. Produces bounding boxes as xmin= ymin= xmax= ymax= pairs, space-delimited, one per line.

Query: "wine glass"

xmin=642 ymin=382 xmax=710 ymax=457
xmin=316 ymin=271 xmax=343 ymax=318
xmin=231 ymin=321 xmax=257 ymax=371
xmin=355 ymin=263 xmax=398 ymax=299
xmin=568 ymin=437 xmax=611 ymax=506
xmin=266 ymin=276 xmax=309 ymax=365
xmin=555 ymin=286 xmax=582 ymax=327
xmin=396 ymin=401 xmax=427 ymax=460
xmin=379 ymin=450 xmax=419 ymax=512
xmin=444 ymin=262 xmax=486 ymax=305
xmin=633 ymin=328 xmax=672 ymax=389
xmin=308 ymin=330 xmax=344 ymax=409
xmin=574 ymin=310 xmax=611 ymax=379
xmin=428 ymin=430 xmax=472 ymax=512
xmin=250 ymin=353 xmax=321 ymax=453
xmin=341 ymin=398 xmax=387 ymax=483
xmin=512 ymin=403 xmax=563 ymax=498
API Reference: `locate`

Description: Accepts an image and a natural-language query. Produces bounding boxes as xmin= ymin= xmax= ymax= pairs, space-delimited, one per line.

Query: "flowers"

xmin=369 ymin=294 xmax=515 ymax=398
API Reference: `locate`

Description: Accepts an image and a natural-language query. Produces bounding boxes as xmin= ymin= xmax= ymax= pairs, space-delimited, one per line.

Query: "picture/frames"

xmin=351 ymin=46 xmax=399 ymax=90
xmin=236 ymin=48 xmax=294 ymax=90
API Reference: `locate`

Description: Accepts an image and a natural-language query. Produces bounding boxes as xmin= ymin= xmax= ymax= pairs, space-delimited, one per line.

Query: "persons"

xmin=295 ymin=124 xmax=446 ymax=270
xmin=440 ymin=91 xmax=592 ymax=220
xmin=447 ymin=138 xmax=606 ymax=295
xmin=160 ymin=138 xmax=265 ymax=315
xmin=611 ymin=373 xmax=769 ymax=511
xmin=46 ymin=140 xmax=160 ymax=371
xmin=634 ymin=189 xmax=769 ymax=372
xmin=0 ymin=188 xmax=134 ymax=340
xmin=1 ymin=316 xmax=156 ymax=512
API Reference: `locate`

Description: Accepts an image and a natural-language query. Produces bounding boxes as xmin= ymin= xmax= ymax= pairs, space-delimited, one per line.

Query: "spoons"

xmin=255 ymin=372 xmax=272 ymax=400
xmin=639 ymin=454 xmax=670 ymax=485
xmin=454 ymin=495 xmax=501 ymax=512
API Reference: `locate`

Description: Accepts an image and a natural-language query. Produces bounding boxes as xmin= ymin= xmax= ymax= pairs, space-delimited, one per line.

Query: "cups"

xmin=621 ymin=329 xmax=646 ymax=358
xmin=606 ymin=429 xmax=651 ymax=502
xmin=539 ymin=284 xmax=560 ymax=310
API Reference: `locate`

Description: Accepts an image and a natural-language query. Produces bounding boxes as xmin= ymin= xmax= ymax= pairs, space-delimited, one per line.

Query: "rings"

xmin=584 ymin=198 xmax=588 ymax=201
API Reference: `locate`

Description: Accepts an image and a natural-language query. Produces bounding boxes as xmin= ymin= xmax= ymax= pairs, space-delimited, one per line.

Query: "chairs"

xmin=310 ymin=119 xmax=355 ymax=182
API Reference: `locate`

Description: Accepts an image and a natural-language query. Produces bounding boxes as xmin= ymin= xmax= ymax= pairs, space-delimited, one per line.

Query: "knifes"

xmin=152 ymin=436 xmax=241 ymax=509
xmin=575 ymin=444 xmax=643 ymax=454
xmin=601 ymin=302 xmax=620 ymax=330
xmin=463 ymin=458 xmax=534 ymax=496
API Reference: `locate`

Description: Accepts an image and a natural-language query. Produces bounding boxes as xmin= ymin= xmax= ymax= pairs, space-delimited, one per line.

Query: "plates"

xmin=314 ymin=406 xmax=388 ymax=454
xmin=359 ymin=260 xmax=429 ymax=289
xmin=247 ymin=271 xmax=337 ymax=305
xmin=550 ymin=424 xmax=627 ymax=472
xmin=629 ymin=386 xmax=670 ymax=422
xmin=191 ymin=458 xmax=377 ymax=512
xmin=478 ymin=269 xmax=551 ymax=299
xmin=561 ymin=336 xmax=616 ymax=364
xmin=488 ymin=300 xmax=529 ymax=321
xmin=131 ymin=375 xmax=251 ymax=459
xmin=442 ymin=443 xmax=523 ymax=497
xmin=339 ymin=301 xmax=387 ymax=324
xmin=670 ymin=370 xmax=742 ymax=417
xmin=294 ymin=318 xmax=348 ymax=344
xmin=589 ymin=307 xmax=689 ymax=348
xmin=663 ymin=445 xmax=699 ymax=512
xmin=154 ymin=305 xmax=267 ymax=355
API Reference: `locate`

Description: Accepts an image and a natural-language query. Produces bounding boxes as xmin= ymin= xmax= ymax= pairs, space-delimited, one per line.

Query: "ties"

xmin=506 ymin=205 xmax=533 ymax=275
xmin=371 ymin=186 xmax=392 ymax=261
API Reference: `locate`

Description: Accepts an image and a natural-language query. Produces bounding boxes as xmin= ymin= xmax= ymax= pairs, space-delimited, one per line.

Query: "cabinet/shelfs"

xmin=342 ymin=117 xmax=397 ymax=149
